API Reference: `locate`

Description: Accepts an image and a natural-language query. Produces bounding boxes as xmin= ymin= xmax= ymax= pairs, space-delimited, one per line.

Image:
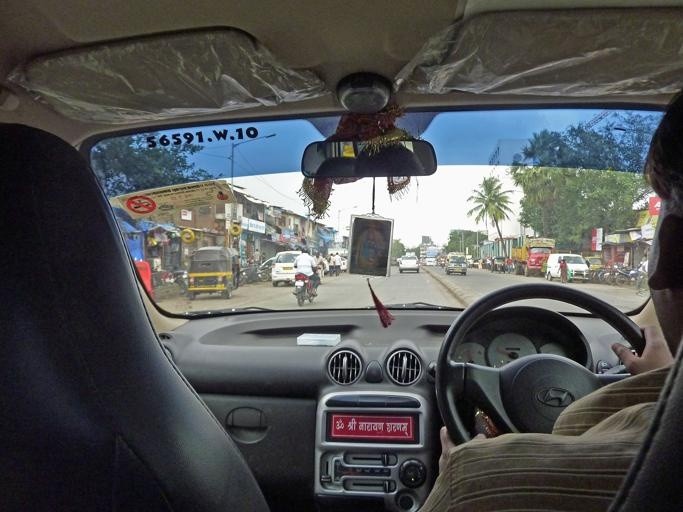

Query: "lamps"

xmin=335 ymin=72 xmax=393 ymax=116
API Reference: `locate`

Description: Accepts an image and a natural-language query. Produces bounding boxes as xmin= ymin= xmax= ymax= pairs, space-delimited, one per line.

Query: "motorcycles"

xmin=238 ymin=259 xmax=269 ymax=286
xmin=294 ymin=267 xmax=320 ymax=306
xmin=590 ymin=262 xmax=643 ymax=287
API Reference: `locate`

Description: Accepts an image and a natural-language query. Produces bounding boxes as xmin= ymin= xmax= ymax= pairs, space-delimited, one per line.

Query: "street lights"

xmin=230 ymin=133 xmax=276 ymax=192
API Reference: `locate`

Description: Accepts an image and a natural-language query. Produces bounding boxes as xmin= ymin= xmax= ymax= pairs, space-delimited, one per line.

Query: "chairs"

xmin=605 ymin=334 xmax=683 ymax=512
xmin=0 ymin=120 xmax=271 ymax=512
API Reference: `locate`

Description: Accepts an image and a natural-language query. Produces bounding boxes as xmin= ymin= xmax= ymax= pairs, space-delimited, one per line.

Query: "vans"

xmin=444 ymin=252 xmax=466 ymax=275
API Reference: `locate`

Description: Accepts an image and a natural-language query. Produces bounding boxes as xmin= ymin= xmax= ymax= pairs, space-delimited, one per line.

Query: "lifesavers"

xmin=180 ymin=229 xmax=195 ymax=243
xmin=229 ymin=224 xmax=242 ymax=236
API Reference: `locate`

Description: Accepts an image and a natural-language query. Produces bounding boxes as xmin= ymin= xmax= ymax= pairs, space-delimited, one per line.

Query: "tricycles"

xmin=489 ymin=256 xmax=504 ymax=274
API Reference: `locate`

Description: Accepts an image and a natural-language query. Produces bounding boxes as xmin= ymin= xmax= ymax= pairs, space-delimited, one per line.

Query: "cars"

xmin=583 ymin=256 xmax=601 ymax=270
xmin=187 ymin=246 xmax=240 ymax=300
xmin=398 ymin=255 xmax=419 ymax=273
xmin=322 ymin=257 xmax=346 ymax=272
xmin=271 ymin=250 xmax=302 ymax=287
xmin=546 ymin=254 xmax=589 ymax=283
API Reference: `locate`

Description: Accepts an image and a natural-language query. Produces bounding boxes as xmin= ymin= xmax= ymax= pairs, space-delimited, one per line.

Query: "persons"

xmin=291 ymin=248 xmax=320 ymax=297
xmin=505 ymin=257 xmax=512 ymax=274
xmin=415 ymin=91 xmax=682 ymax=511
xmin=556 ymin=260 xmax=568 ymax=283
xmin=628 ymin=255 xmax=645 ymax=291
xmin=245 ymin=245 xmax=342 ymax=277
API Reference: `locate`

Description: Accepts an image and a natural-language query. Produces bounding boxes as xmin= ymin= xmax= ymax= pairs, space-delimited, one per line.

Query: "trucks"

xmin=511 ymin=237 xmax=555 ymax=277
